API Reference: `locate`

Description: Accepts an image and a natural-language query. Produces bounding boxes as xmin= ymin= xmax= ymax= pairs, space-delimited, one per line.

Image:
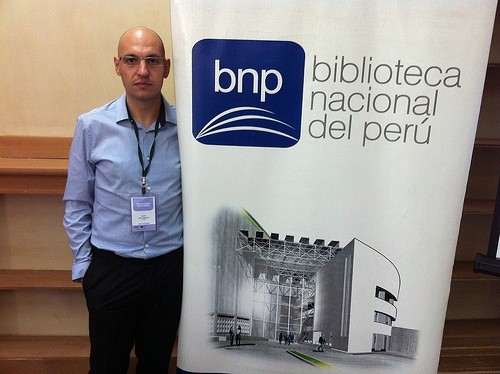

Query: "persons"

xmin=317 ymin=333 xmax=334 ymax=352
xmin=63 ymin=26 xmax=184 ymax=374
xmin=279 ymin=330 xmax=295 ymax=345
xmin=229 ymin=325 xmax=242 ymax=349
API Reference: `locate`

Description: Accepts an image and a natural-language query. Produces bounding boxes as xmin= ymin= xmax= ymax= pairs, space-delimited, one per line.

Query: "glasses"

xmin=118 ymin=55 xmax=167 ymax=68
